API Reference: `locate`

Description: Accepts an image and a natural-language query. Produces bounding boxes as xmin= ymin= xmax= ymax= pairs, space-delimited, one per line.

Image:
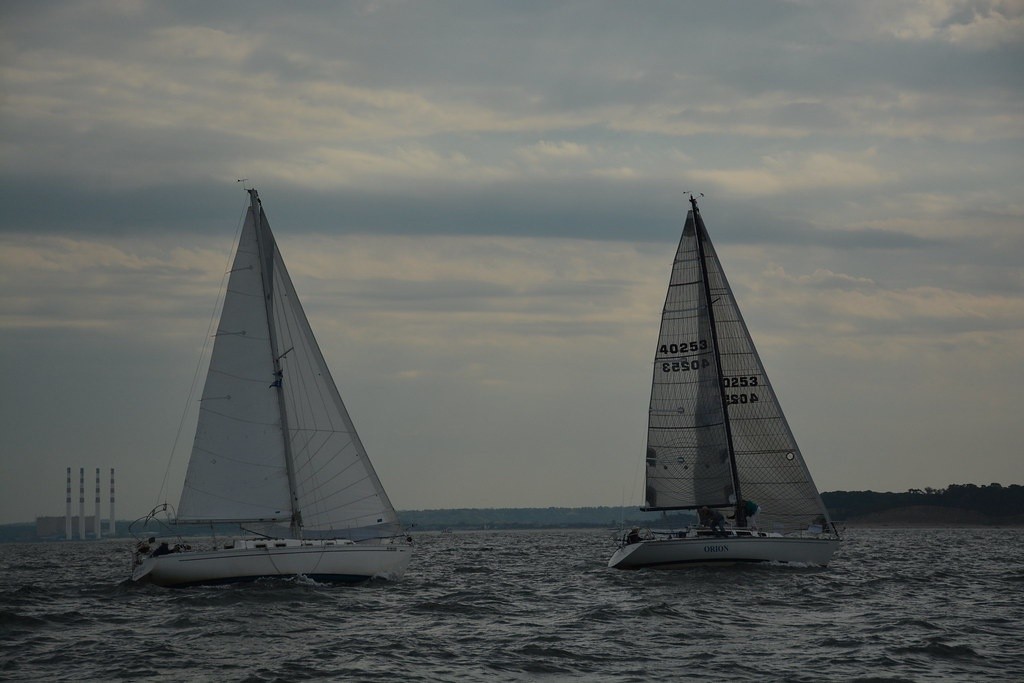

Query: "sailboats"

xmin=129 ymin=177 xmax=415 ymax=585
xmin=608 ymin=189 xmax=842 ymax=569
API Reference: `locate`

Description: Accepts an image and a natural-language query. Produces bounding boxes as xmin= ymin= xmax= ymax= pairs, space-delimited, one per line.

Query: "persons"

xmin=150 ymin=542 xmax=177 ymax=558
xmin=742 ymin=499 xmax=761 ymax=530
xmin=702 ymin=506 xmax=727 ymax=538
xmin=628 ymin=527 xmax=643 ymax=544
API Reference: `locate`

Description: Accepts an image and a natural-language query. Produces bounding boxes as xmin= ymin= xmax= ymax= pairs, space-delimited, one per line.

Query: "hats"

xmin=703 ymin=506 xmax=707 ymax=512
xmin=632 ymin=525 xmax=639 ymax=529
xmin=161 ymin=542 xmax=168 ymax=544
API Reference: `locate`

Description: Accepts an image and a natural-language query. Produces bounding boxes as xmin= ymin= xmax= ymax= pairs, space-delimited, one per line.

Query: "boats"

xmin=880 ymin=524 xmax=890 ymax=527
xmin=441 ymin=526 xmax=454 ymax=534
